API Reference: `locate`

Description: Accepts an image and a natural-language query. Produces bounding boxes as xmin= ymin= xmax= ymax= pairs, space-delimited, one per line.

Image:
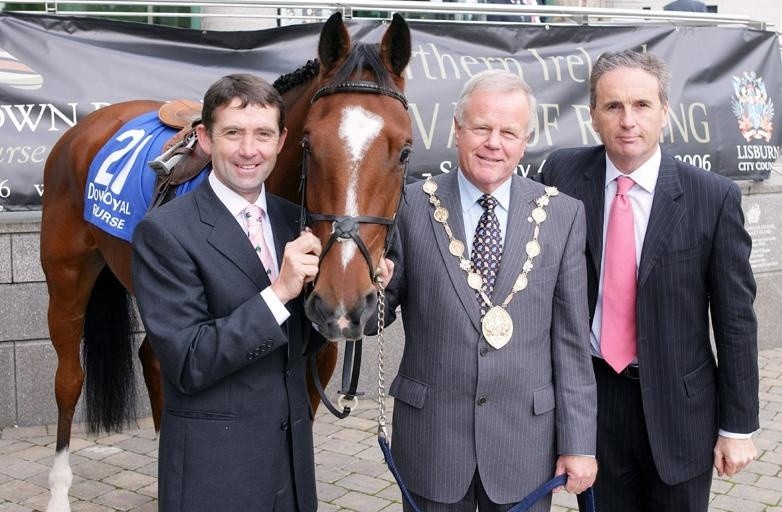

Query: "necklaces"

xmin=425 ymin=174 xmax=557 ymax=350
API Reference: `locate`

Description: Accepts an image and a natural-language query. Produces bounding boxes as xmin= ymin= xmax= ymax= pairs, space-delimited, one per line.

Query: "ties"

xmin=241 ymin=209 xmax=276 ymax=282
xmin=469 ymin=195 xmax=502 ymax=323
xmin=600 ymin=176 xmax=638 ymax=374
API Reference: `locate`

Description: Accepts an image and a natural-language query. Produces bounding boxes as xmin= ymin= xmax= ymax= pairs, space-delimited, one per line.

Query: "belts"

xmin=625 ymin=364 xmax=640 ymax=380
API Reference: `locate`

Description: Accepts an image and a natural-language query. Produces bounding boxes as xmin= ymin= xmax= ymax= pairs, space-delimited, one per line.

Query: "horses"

xmin=38 ymin=11 xmax=413 ymax=512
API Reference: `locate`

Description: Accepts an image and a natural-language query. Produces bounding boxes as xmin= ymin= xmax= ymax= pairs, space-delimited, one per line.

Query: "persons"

xmin=133 ymin=73 xmax=327 ymax=510
xmin=404 ymin=51 xmax=759 ymax=511
xmin=365 ymin=69 xmax=600 ymax=510
